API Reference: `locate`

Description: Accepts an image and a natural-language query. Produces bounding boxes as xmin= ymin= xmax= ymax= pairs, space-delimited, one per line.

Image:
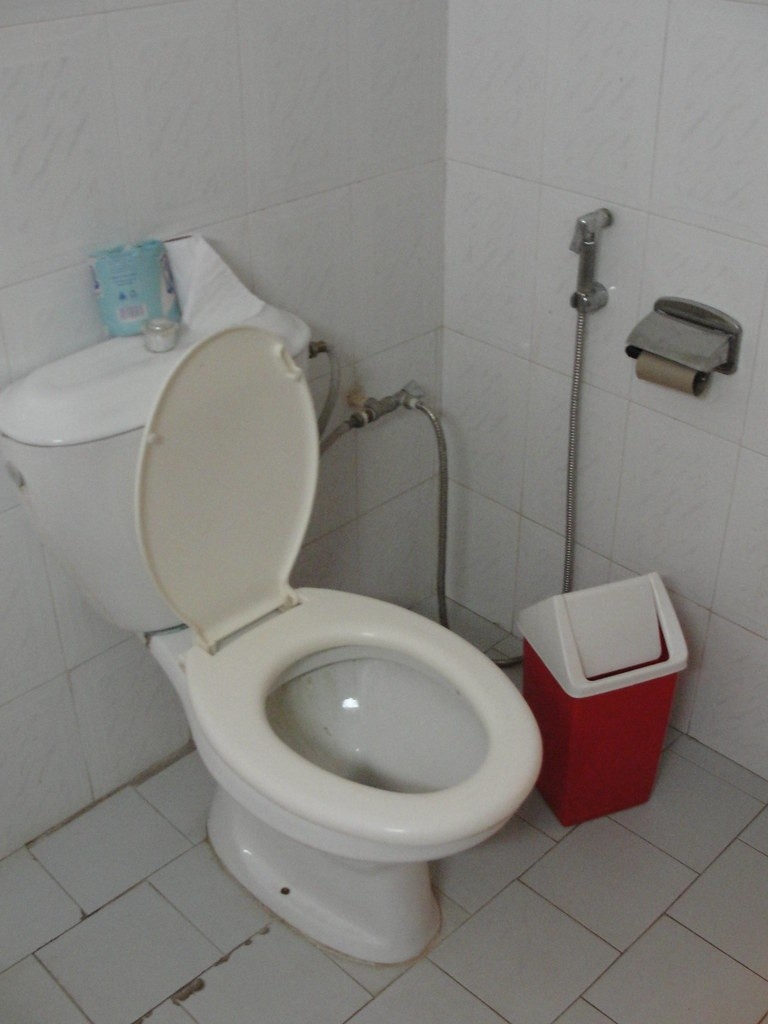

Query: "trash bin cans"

xmin=515 ymin=572 xmax=688 ymax=826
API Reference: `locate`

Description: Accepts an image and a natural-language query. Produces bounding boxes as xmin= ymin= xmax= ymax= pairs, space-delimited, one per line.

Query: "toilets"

xmin=0 ymin=321 xmax=507 ymax=957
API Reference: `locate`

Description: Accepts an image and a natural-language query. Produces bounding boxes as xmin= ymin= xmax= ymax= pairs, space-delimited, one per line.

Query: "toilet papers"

xmin=161 ymin=231 xmax=266 ymax=350
